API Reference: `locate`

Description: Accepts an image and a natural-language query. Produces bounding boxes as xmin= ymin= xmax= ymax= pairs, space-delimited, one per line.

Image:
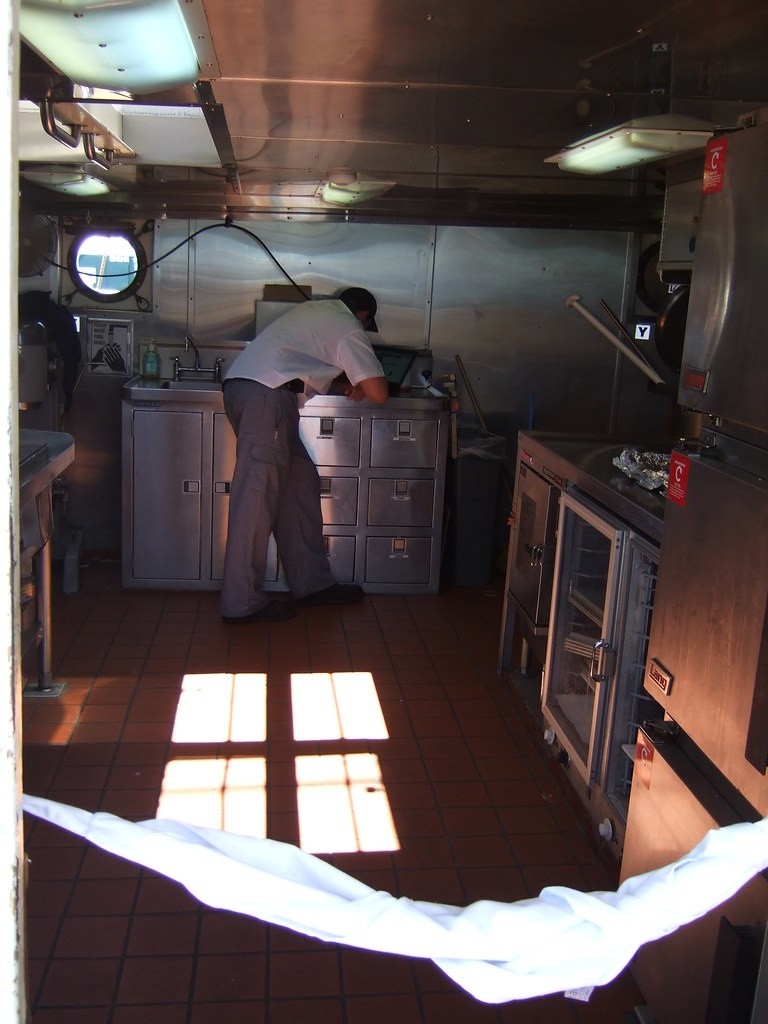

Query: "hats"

xmin=339 ymin=287 xmax=379 ymax=333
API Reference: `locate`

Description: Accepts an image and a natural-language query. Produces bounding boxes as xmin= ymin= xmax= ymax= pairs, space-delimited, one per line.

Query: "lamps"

xmin=544 ymin=114 xmax=713 ymax=174
xmin=312 ymin=178 xmax=396 ymax=205
xmin=18 ymin=164 xmax=119 ymax=196
xmin=15 ymin=1 xmax=221 ymax=95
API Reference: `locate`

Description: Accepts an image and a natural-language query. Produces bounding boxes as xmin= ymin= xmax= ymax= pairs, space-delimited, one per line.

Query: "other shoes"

xmin=295 ymin=583 xmax=365 ymax=605
xmin=221 ymin=600 xmax=297 ymax=623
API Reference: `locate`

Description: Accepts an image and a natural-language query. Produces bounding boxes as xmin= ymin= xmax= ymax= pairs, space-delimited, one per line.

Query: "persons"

xmin=221 ymin=288 xmax=390 ymax=623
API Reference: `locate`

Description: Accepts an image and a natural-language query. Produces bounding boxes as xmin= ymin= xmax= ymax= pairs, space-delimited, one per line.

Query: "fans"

xmin=19 ymin=208 xmax=58 ymax=279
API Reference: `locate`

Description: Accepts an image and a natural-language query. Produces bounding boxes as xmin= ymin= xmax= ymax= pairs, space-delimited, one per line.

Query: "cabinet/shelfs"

xmin=121 ymin=410 xmax=450 ymax=593
xmin=508 ymin=462 xmax=559 ymax=637
